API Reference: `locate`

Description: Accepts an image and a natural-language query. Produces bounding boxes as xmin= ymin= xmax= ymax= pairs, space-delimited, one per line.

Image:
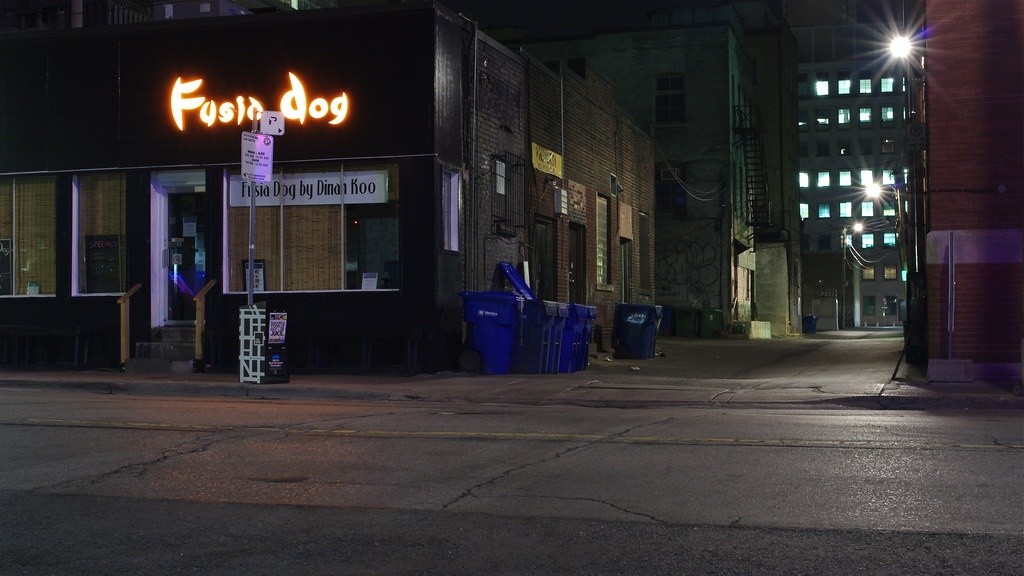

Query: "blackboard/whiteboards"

xmin=84 ymin=233 xmax=121 ymax=293
xmin=0 ymin=239 xmax=11 ymax=295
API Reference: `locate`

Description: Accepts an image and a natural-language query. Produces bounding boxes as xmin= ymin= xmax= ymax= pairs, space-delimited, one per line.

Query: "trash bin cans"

xmin=238 ymin=300 xmax=290 ymax=384
xmin=459 ymin=262 xmax=597 ymax=374
xmin=611 ymin=302 xmax=663 ymax=359
xmin=658 ymin=305 xmax=724 ymax=338
xmin=805 ymin=314 xmax=818 ymax=334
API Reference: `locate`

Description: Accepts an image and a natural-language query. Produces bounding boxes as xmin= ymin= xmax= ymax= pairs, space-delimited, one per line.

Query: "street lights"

xmin=864 ymin=182 xmax=908 ymax=300
xmin=842 ymin=223 xmax=863 ymax=330
